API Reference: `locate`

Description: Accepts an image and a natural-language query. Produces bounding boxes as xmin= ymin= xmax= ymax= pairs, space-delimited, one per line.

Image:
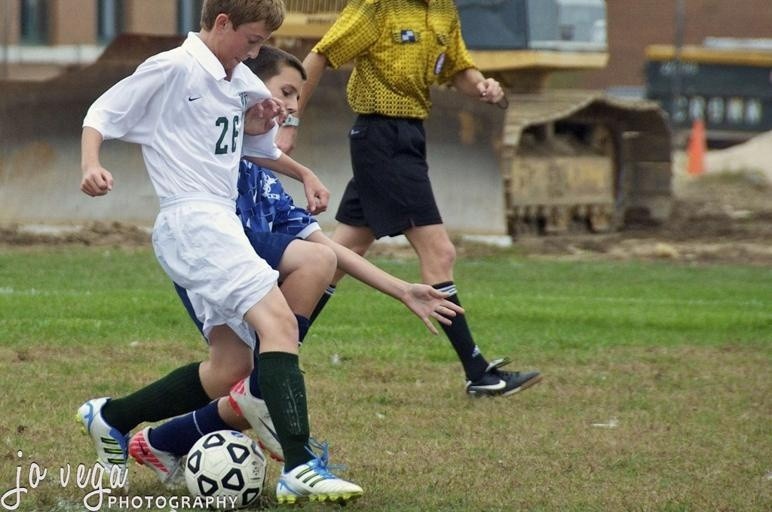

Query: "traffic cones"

xmin=685 ymin=119 xmax=707 ymax=172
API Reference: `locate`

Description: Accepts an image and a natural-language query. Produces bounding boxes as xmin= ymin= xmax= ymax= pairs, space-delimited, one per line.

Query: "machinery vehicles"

xmin=0 ymin=0 xmax=676 ymax=253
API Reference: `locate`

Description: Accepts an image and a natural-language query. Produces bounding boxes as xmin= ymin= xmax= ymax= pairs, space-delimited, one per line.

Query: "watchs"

xmin=282 ymin=113 xmax=300 ymax=126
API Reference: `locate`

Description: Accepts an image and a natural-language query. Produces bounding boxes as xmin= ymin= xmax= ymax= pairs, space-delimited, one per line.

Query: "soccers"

xmin=185 ymin=429 xmax=267 ymax=511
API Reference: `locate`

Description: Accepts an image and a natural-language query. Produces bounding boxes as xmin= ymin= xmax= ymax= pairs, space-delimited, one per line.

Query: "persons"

xmin=128 ymin=44 xmax=465 ymax=491
xmin=276 ymin=0 xmax=545 ymax=398
xmin=74 ymin=0 xmax=364 ymax=507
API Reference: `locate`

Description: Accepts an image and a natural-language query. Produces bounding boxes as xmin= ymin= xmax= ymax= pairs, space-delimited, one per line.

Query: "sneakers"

xmin=75 ymin=396 xmax=132 ymax=495
xmin=128 ymin=427 xmax=186 ymax=491
xmin=228 ymin=376 xmax=287 ymax=462
xmin=465 ymin=356 xmax=545 ymax=400
xmin=274 ymin=435 xmax=366 ymax=506
